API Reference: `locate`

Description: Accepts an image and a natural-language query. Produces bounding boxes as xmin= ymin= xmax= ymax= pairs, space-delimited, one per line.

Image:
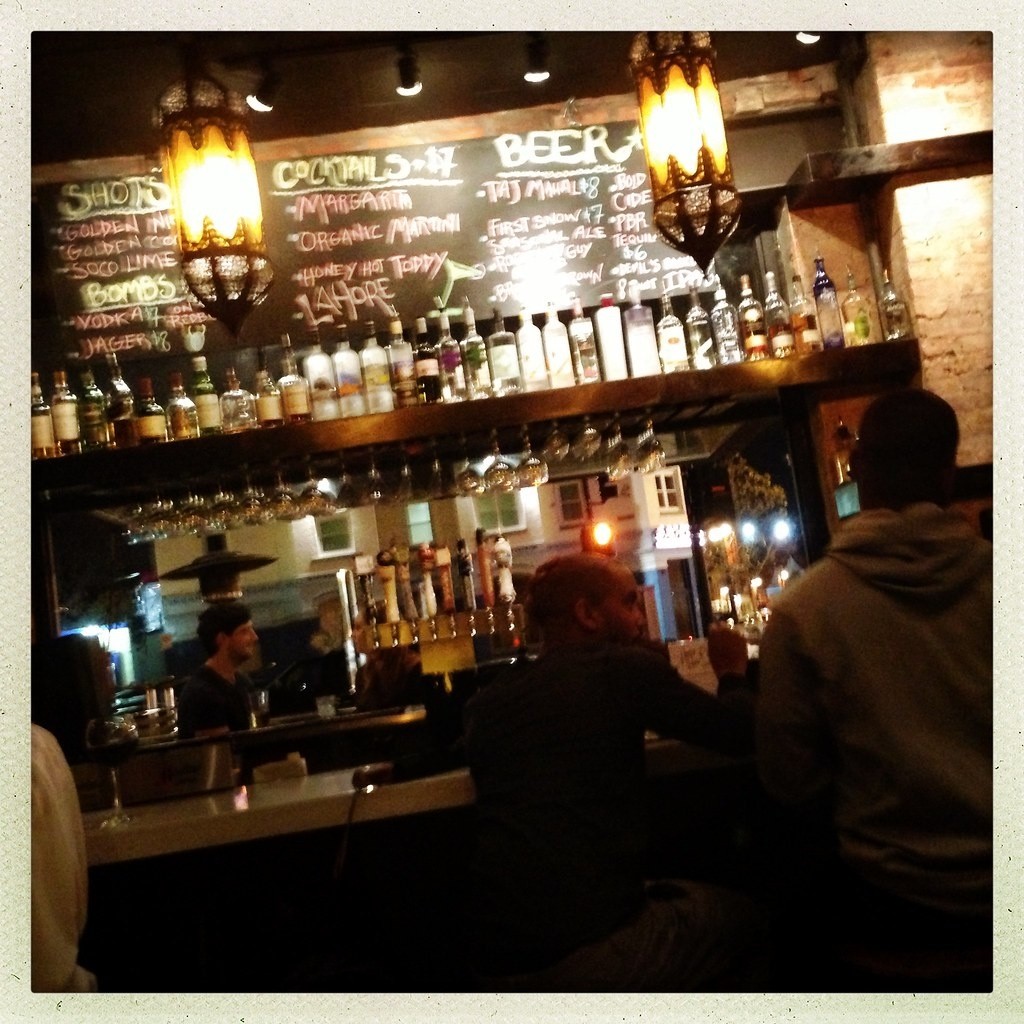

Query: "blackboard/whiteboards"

xmin=31 ymin=120 xmax=719 ymax=373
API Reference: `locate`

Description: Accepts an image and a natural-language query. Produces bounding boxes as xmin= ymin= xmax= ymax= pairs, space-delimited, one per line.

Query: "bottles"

xmin=30 ymin=253 xmax=918 ymax=465
xmin=831 ymin=425 xmax=860 ymax=485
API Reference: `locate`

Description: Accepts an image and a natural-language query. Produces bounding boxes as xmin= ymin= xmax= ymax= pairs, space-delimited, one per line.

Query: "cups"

xmin=247 ymin=690 xmax=271 ymax=728
xmin=316 ymin=694 xmax=335 ymax=720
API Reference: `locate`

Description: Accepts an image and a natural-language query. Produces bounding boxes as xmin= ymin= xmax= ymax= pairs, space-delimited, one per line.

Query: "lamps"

xmin=522 ymin=39 xmax=551 ymax=84
xmin=396 ymin=56 xmax=422 ymax=98
xmin=629 ymin=31 xmax=741 ymax=275
xmin=150 ymin=63 xmax=282 ymax=335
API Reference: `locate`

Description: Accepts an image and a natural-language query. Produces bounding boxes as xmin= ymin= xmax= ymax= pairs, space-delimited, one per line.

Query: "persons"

xmin=764 ymin=390 xmax=994 ymax=993
xmin=177 ymin=604 xmax=258 ymax=735
xmin=351 ymin=607 xmax=452 ymax=783
xmin=30 ymin=724 xmax=101 ymax=993
xmin=459 ymin=555 xmax=755 ymax=992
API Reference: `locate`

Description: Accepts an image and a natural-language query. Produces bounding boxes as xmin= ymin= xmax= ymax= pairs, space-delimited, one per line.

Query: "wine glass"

xmin=123 ymin=408 xmax=668 ymax=550
xmin=85 ymin=713 xmax=142 ymax=831
